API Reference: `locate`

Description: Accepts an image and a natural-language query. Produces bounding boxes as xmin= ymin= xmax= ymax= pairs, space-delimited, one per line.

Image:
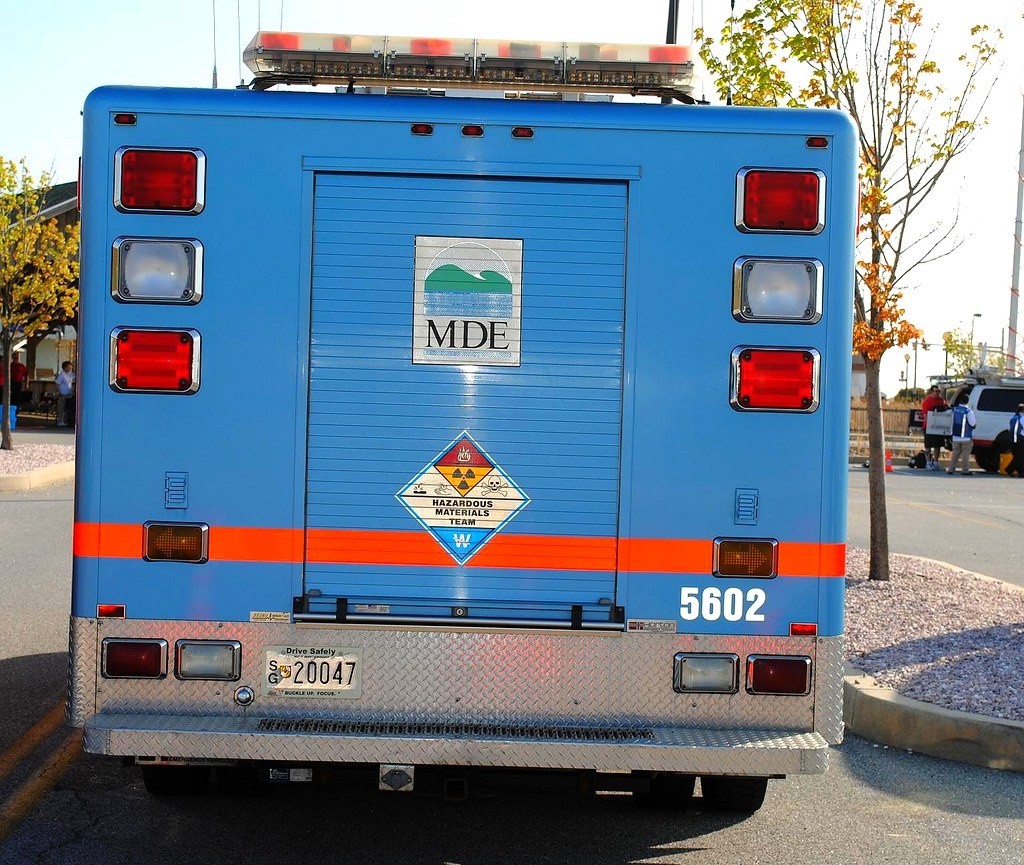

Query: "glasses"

xmin=932 ymin=390 xmax=941 ymax=393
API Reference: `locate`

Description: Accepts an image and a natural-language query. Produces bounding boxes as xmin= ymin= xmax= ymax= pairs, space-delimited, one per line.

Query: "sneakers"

xmin=926 ymin=463 xmax=947 ymax=472
xmin=58 ymin=422 xmax=65 ymax=426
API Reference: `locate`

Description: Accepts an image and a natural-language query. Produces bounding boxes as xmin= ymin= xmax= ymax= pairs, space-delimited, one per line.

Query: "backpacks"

xmin=908 ymin=450 xmax=926 ymax=468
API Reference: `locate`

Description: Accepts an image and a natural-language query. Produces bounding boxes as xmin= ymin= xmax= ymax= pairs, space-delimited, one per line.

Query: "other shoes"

xmin=948 ymin=471 xmax=954 ymax=476
xmin=961 ymin=471 xmax=975 ymax=476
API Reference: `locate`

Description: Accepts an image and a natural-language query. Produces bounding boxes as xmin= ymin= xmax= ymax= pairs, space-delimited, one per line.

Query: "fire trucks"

xmin=67 ymin=26 xmax=859 ymax=825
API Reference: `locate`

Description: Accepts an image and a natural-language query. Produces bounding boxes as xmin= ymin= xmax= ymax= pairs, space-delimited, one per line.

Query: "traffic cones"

xmin=885 ymin=447 xmax=894 ymax=473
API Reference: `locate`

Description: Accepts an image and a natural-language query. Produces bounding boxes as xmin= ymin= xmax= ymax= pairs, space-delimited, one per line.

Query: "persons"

xmin=10 ymin=352 xmax=29 ymax=414
xmin=55 ymin=361 xmax=76 ymax=427
xmin=922 ymin=386 xmax=946 ymax=471
xmin=947 ymin=395 xmax=976 ymax=474
xmin=1004 ymin=403 xmax=1024 ymax=478
xmin=0 ymin=355 xmax=4 ymax=404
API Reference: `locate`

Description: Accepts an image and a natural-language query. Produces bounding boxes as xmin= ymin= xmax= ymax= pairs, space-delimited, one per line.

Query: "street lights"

xmin=967 ymin=312 xmax=981 ymax=375
xmin=899 ymin=352 xmax=910 ymax=392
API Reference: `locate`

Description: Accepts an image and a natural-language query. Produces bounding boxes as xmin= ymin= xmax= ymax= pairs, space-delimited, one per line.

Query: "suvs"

xmin=925 ymin=364 xmax=1024 ymax=473
